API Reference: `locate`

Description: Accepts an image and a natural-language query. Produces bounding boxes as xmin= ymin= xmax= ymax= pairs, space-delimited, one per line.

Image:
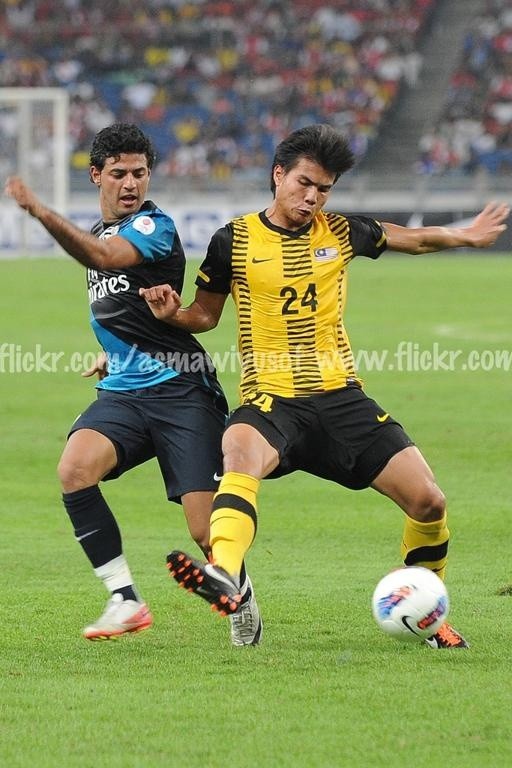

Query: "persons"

xmin=5 ymin=123 xmax=263 ymax=648
xmin=139 ymin=122 xmax=510 ymax=650
xmin=1 ymin=2 xmax=511 ymax=182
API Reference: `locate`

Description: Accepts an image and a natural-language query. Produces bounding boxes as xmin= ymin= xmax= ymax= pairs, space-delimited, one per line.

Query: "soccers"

xmin=372 ymin=569 xmax=450 ymax=639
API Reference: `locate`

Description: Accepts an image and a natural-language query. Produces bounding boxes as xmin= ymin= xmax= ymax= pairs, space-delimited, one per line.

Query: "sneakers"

xmin=228 ymin=574 xmax=261 ymax=645
xmin=425 ymin=622 xmax=469 ymax=649
xmin=167 ymin=551 xmax=242 ymax=616
xmin=84 ymin=594 xmax=153 ymax=641
